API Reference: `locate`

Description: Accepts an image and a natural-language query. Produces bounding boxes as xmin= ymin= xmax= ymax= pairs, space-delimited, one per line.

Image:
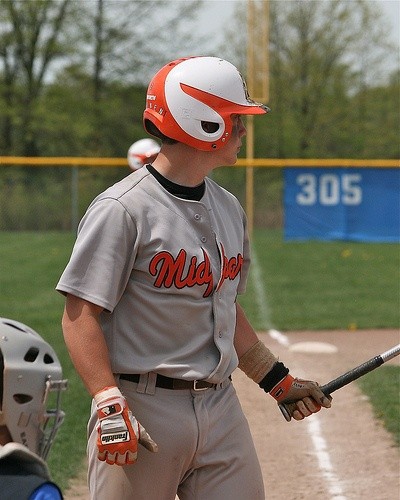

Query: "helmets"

xmin=0 ymin=317 xmax=68 ymax=462
xmin=127 ymin=138 xmax=160 ymax=170
xmin=143 ymin=56 xmax=271 ymax=151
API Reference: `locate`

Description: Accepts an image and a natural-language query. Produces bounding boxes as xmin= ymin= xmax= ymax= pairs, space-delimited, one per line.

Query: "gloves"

xmin=91 ymin=386 xmax=159 ymax=467
xmin=259 ymin=363 xmax=332 ymax=421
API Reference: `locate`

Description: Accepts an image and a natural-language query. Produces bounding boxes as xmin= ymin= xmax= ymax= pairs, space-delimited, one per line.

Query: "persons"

xmin=54 ymin=56 xmax=333 ymax=500
xmin=0 ymin=317 xmax=70 ymax=500
xmin=126 ymin=138 xmax=162 ymax=176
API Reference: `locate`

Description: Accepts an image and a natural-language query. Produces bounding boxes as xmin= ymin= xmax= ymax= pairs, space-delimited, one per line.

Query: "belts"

xmin=120 ymin=372 xmax=214 ymax=391
